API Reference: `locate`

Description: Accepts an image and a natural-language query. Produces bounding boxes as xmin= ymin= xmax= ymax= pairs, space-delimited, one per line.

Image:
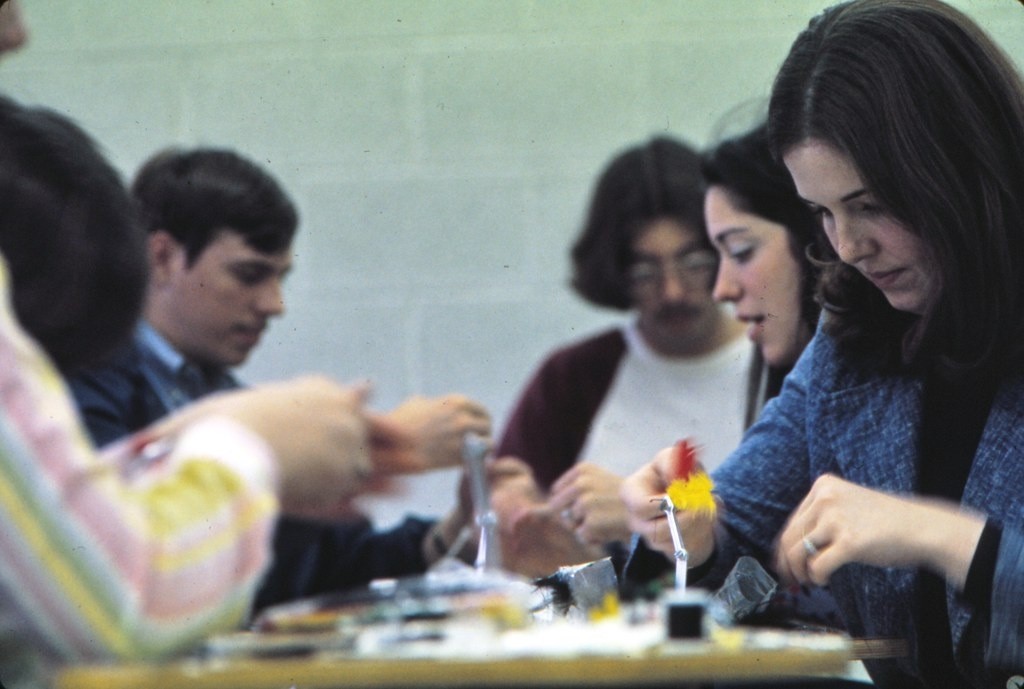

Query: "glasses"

xmin=629 ymin=251 xmax=716 ymax=293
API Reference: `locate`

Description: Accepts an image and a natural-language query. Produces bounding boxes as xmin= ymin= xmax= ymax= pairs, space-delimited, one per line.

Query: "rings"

xmin=802 ymin=536 xmax=818 ymax=555
xmin=561 ymin=502 xmax=583 ymax=525
xmin=354 ymin=453 xmax=375 ymax=481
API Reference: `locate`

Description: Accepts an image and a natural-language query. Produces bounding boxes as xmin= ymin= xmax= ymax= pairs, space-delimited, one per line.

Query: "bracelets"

xmin=433 ymin=523 xmax=465 ymax=559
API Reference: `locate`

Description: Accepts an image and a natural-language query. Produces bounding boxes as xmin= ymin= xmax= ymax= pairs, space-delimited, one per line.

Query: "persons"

xmin=0 ymin=0 xmax=429 ymax=688
xmin=461 ymin=133 xmax=793 ymax=580
xmin=498 ymin=119 xmax=849 ymax=635
xmin=621 ymin=0 xmax=1024 ymax=688
xmin=67 ymin=143 xmax=494 ymax=623
